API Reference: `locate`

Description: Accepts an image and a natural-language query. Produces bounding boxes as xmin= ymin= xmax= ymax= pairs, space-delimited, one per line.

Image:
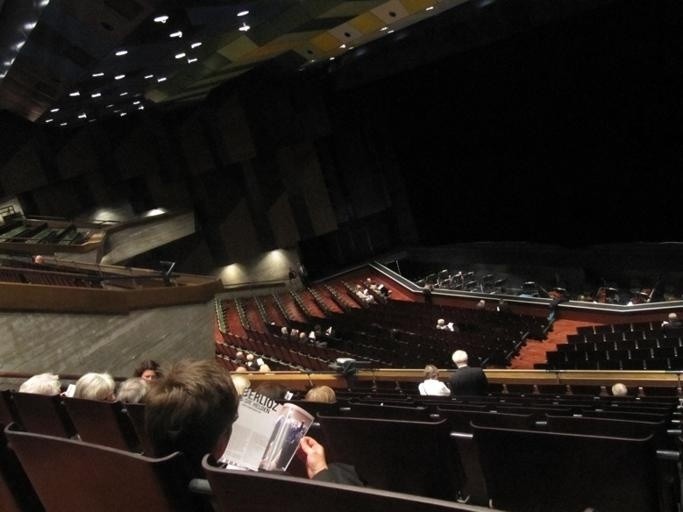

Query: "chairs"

xmin=0 ymin=213 xmax=97 ymax=289
xmin=214 ymin=266 xmax=683 ymax=371
xmin=1 ymin=382 xmax=683 ymax=511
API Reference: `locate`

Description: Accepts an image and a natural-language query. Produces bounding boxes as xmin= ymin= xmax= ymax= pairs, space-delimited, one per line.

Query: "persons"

xmin=611 ymin=383 xmax=628 ymax=398
xmin=661 ymin=312 xmax=682 ymax=328
